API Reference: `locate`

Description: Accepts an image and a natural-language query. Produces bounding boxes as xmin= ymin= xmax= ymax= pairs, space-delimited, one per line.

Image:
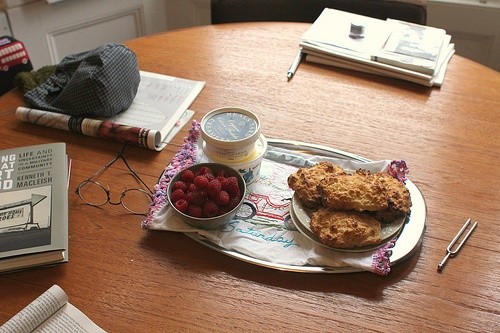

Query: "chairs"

xmin=210 ymin=0 xmax=428 ymax=26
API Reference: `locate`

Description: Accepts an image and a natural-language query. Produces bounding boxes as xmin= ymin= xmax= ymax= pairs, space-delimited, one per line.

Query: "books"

xmin=16 ymin=70 xmax=206 ymax=151
xmin=0 ymin=284 xmax=108 ymax=333
xmin=300 ymin=8 xmax=455 ymax=87
xmin=0 ymin=141 xmax=73 ymax=274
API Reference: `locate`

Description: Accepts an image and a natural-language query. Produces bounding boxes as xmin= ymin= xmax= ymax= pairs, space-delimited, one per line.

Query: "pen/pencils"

xmin=287 ymin=48 xmax=304 ymax=78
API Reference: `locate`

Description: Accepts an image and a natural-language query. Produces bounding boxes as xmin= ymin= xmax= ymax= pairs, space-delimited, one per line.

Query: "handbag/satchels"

xmin=15 ymin=42 xmax=141 ymax=117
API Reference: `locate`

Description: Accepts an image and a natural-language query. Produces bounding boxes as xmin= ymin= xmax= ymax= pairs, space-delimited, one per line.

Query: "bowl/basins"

xmin=167 ymin=162 xmax=247 ymax=230
xmin=199 ymin=107 xmax=261 ymax=162
xmin=202 ymin=133 xmax=268 ymax=186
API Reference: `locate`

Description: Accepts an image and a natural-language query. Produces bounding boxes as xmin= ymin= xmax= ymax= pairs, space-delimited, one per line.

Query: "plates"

xmin=289 ymin=169 xmax=406 ymax=252
xmin=158 ymin=138 xmax=427 ymax=276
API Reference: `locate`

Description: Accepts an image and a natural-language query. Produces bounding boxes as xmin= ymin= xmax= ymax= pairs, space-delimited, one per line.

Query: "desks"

xmin=0 ymin=23 xmax=500 ymax=333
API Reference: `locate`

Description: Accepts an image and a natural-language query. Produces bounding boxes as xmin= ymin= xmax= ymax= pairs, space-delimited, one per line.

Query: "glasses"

xmin=74 ymin=139 xmax=154 ymax=215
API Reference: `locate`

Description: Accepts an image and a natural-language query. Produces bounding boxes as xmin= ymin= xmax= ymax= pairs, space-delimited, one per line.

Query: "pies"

xmin=288 ymin=162 xmax=413 ymax=249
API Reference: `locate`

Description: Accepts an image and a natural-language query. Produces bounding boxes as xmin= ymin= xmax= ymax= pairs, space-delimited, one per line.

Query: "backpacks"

xmin=0 ymin=35 xmax=33 ymax=98
